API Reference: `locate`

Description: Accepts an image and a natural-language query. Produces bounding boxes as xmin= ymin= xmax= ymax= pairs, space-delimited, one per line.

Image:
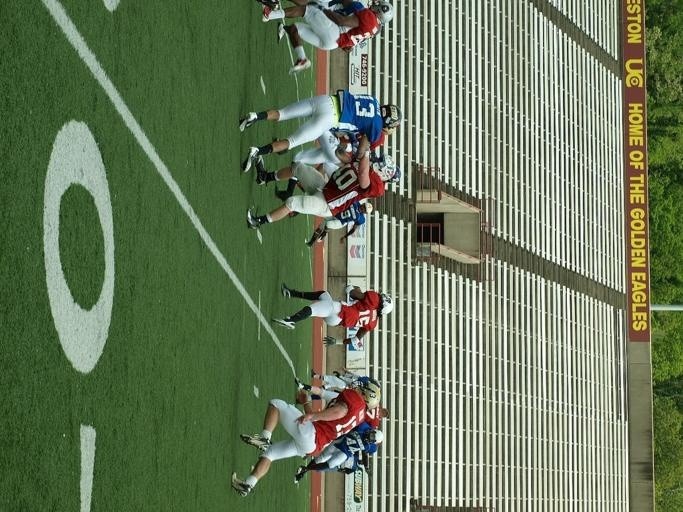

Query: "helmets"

xmin=372 ymin=105 xmax=403 ymax=184
xmin=380 ymin=294 xmax=395 ymax=315
xmin=362 ymin=200 xmax=372 ymax=215
xmin=352 ymin=376 xmax=382 ymax=445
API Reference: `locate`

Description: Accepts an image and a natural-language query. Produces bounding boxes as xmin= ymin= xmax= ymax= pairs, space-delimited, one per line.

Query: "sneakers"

xmin=271 ymin=283 xmax=295 ymax=329
xmin=259 ymin=3 xmax=311 ymax=74
xmin=306 ymin=226 xmax=327 ymax=247
xmin=231 ymin=434 xmax=272 ymax=497
xmin=240 ymin=109 xmax=298 ymax=229
xmin=294 ymin=369 xmax=326 ymax=484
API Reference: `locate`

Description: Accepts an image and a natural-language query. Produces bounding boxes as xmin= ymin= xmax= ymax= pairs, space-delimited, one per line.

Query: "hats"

xmin=372 ymin=0 xmax=394 ymax=24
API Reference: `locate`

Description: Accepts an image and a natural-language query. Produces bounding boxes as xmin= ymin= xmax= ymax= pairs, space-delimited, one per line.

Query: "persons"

xmin=272 ymin=281 xmax=394 ymax=347
xmin=231 ymin=366 xmax=389 ymax=498
xmin=239 ymin=89 xmax=399 ymax=231
xmin=260 ymin=1 xmax=394 ymax=73
xmin=305 ymin=202 xmax=373 ymax=247
